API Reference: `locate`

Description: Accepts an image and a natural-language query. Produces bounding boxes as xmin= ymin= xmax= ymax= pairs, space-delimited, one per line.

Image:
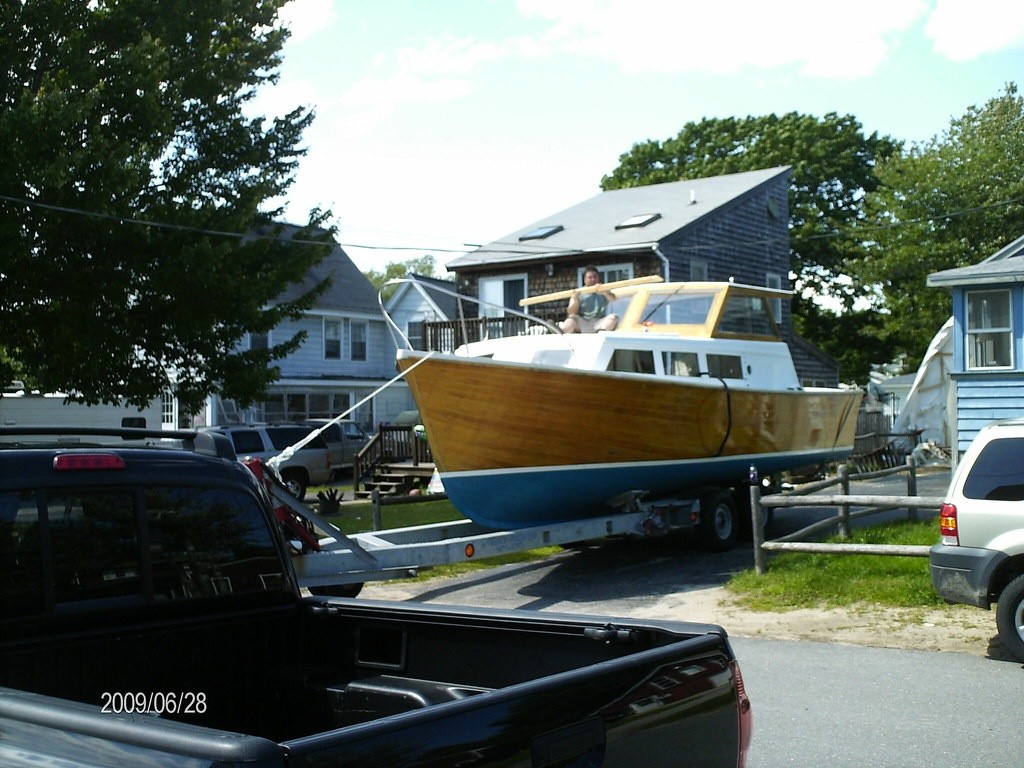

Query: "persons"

xmin=547 ymin=265 xmax=619 ymax=334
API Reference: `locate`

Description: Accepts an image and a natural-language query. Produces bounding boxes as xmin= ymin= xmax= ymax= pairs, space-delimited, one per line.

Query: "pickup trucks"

xmin=305 ymin=417 xmax=379 ymax=472
xmin=0 ymin=424 xmax=754 ymax=768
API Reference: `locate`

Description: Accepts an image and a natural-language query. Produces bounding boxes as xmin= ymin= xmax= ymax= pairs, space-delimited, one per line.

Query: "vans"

xmin=178 ymin=420 xmax=333 ymax=501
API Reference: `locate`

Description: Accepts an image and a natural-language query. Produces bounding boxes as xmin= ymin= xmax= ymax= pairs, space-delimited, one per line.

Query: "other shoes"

xmin=545 ymin=319 xmax=563 ymax=334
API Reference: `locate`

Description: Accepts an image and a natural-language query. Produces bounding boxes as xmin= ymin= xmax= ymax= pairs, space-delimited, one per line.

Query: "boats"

xmin=377 ymin=275 xmax=865 ymax=535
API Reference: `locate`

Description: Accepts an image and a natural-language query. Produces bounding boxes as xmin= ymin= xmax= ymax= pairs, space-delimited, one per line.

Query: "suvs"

xmin=927 ymin=418 xmax=1024 ymax=663
